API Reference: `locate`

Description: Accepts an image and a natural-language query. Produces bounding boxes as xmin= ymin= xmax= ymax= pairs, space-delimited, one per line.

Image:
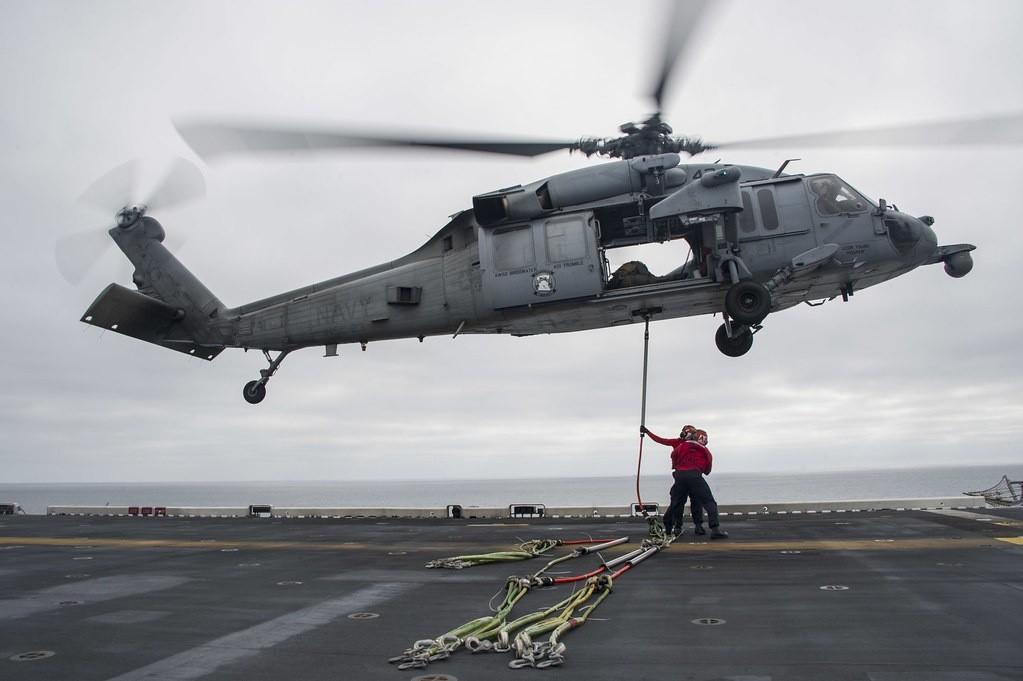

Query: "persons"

xmin=812 ymin=179 xmax=845 ymax=213
xmin=640 ymin=425 xmax=729 ymax=540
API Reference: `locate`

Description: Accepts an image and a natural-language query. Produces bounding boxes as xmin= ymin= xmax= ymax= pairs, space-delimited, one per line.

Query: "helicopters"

xmin=76 ymin=0 xmax=975 ymax=403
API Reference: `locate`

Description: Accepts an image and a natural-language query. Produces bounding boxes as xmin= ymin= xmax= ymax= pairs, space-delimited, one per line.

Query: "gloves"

xmin=640 ymin=425 xmax=649 ymax=433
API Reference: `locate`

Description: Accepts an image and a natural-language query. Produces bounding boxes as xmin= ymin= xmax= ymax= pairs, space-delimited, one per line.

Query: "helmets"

xmin=693 ymin=430 xmax=707 ymax=445
xmin=681 ymin=426 xmax=696 ymax=439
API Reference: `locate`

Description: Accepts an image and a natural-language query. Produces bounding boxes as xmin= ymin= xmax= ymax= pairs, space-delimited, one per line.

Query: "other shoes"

xmin=663 ymin=528 xmax=671 ymax=535
xmin=674 ymin=526 xmax=681 ymax=536
xmin=709 ymin=527 xmax=729 ymax=538
xmin=694 ymin=525 xmax=707 ymax=534
